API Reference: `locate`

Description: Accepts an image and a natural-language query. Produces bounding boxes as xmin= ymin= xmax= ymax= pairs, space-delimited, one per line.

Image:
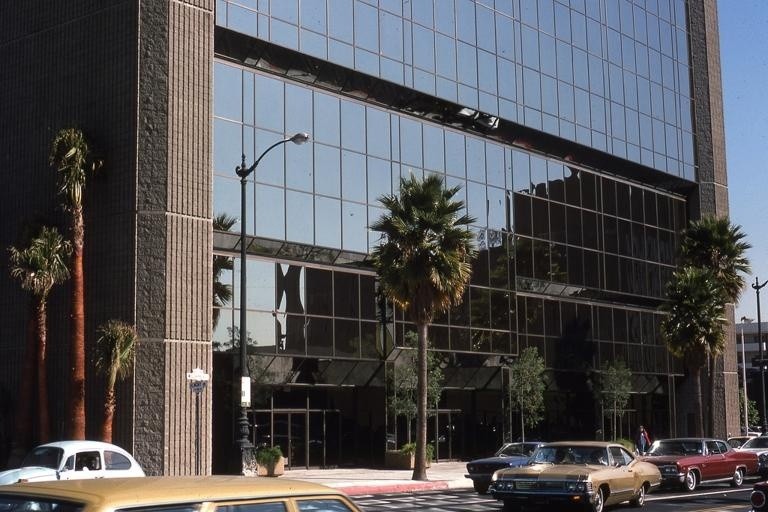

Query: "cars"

xmin=726 ymin=436 xmax=767 ymax=456
xmin=636 ymin=437 xmax=760 ymax=493
xmin=463 ymin=441 xmax=545 ymax=495
xmin=1 ymin=440 xmax=147 ymax=484
xmin=255 ymin=419 xmax=302 ymax=467
xmin=747 ymin=479 xmax=768 ymax=512
xmin=487 ymin=440 xmax=663 ymax=512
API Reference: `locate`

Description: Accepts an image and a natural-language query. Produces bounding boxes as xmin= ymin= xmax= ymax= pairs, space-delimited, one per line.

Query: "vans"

xmin=0 ymin=473 xmax=367 ymax=512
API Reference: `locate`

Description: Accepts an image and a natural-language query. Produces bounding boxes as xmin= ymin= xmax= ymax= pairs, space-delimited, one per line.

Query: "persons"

xmin=635 ymin=424 xmax=652 ymax=455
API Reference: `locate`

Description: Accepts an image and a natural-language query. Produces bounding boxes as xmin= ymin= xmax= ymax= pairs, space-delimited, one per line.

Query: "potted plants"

xmin=385 ymin=441 xmax=434 ymax=469
xmin=254 ymin=444 xmax=284 ymax=477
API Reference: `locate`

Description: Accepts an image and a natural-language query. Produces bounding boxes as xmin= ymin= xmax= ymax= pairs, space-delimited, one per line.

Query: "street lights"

xmin=739 ymin=316 xmax=756 ymax=437
xmin=233 ymin=129 xmax=310 ymax=446
